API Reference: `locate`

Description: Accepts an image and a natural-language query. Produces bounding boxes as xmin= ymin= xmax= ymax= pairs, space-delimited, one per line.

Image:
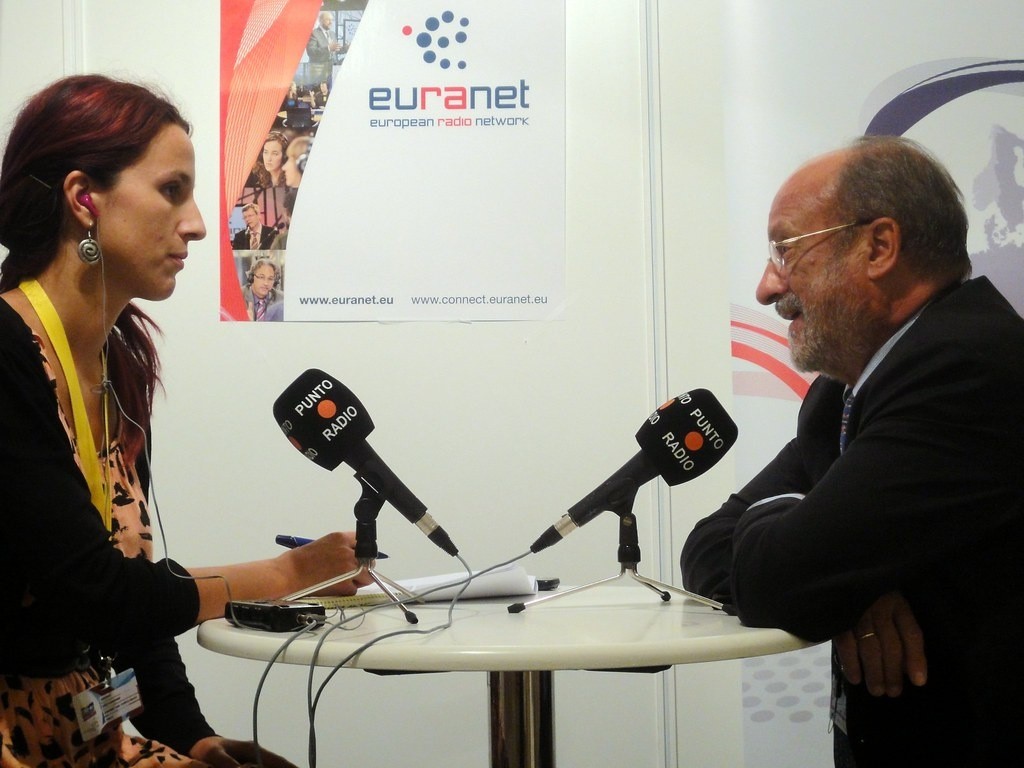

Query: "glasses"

xmin=768 ymin=220 xmax=872 ymax=272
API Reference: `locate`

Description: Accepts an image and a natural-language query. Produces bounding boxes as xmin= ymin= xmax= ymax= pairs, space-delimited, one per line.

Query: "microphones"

xmin=531 ymin=389 xmax=739 ymax=552
xmin=273 ymin=369 xmax=459 ymax=557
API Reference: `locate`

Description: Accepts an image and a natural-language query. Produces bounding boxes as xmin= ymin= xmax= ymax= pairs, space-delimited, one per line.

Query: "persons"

xmin=233 ymin=11 xmax=342 ymax=321
xmin=676 ymin=140 xmax=1022 ymax=767
xmin=3 ymin=75 xmax=376 ymax=765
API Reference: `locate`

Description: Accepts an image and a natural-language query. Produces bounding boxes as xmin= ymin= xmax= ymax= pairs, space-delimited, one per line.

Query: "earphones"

xmin=79 ymin=194 xmax=101 ymax=221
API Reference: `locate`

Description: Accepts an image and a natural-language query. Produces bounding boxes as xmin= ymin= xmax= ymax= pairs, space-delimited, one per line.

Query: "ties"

xmin=840 ymin=392 xmax=855 ymax=454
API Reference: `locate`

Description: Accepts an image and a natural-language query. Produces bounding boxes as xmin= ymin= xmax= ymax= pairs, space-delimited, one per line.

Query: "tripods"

xmin=507 ymin=501 xmax=737 ymax=617
xmin=277 ymin=472 xmax=426 ymax=623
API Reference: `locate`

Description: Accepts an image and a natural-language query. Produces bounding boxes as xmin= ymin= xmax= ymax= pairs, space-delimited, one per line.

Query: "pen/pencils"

xmin=274 ymin=535 xmax=390 ymax=562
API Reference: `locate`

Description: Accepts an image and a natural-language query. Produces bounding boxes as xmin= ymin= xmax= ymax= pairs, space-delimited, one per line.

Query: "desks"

xmin=198 ymin=582 xmax=825 ymax=768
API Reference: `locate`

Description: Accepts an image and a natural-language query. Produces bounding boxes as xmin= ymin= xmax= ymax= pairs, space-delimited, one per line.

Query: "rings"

xmin=859 ymin=632 xmax=875 ymax=640
